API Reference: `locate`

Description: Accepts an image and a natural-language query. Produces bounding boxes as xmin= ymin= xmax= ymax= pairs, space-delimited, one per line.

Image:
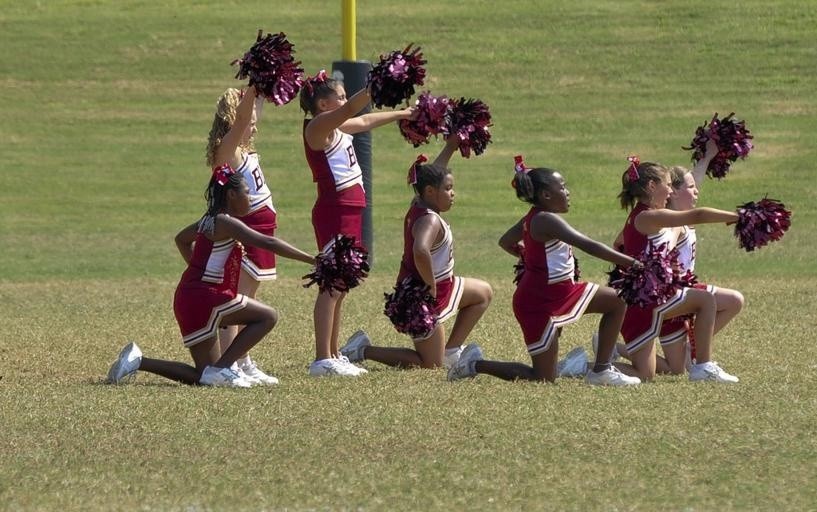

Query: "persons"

xmin=339 ymin=125 xmax=494 ymax=371
xmin=299 ymin=73 xmax=428 ymax=378
xmin=445 ymin=167 xmax=649 ymax=386
xmin=592 ymin=136 xmax=744 ymax=376
xmin=556 ymin=161 xmax=765 ymax=384
xmin=105 ymin=170 xmax=342 ymax=388
xmin=206 ymin=63 xmax=279 ymax=386
